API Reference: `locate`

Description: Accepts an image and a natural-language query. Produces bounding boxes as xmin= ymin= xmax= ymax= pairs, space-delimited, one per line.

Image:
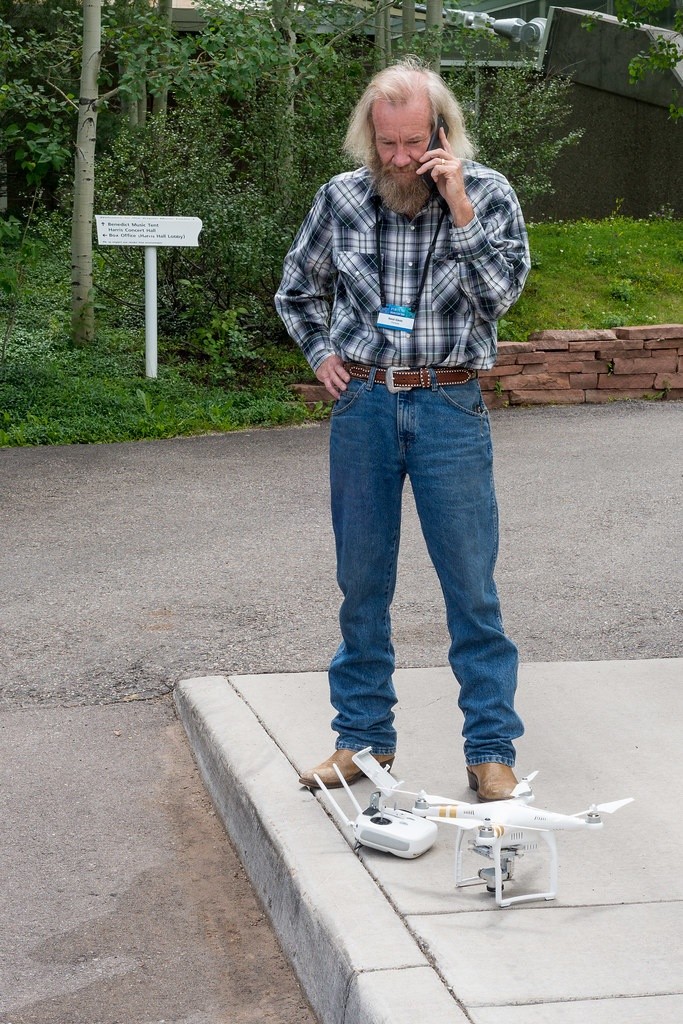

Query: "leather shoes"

xmin=466 ymin=762 xmax=519 ymax=802
xmin=299 ymin=749 xmax=395 ymax=788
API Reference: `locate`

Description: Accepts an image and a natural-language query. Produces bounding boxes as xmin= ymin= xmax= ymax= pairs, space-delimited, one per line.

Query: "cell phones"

xmin=421 ymin=113 xmax=449 ymax=191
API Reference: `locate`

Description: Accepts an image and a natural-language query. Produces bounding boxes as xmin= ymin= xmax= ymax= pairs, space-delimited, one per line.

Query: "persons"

xmin=274 ymin=58 xmax=532 ymax=800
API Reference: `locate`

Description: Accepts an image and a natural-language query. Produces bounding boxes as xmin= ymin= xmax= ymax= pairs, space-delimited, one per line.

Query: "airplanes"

xmin=374 ymin=767 xmax=636 ymax=909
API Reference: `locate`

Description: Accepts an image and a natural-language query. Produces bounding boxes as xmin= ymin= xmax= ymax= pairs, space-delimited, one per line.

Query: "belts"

xmin=343 ymin=363 xmax=478 ymax=394
